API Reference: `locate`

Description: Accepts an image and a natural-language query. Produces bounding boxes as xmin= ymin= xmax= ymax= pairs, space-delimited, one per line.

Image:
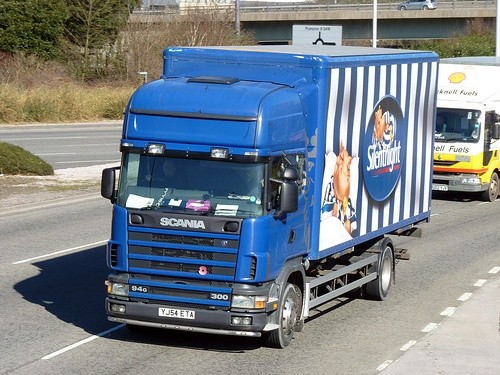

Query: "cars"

xmin=397 ymin=0 xmax=438 ymax=11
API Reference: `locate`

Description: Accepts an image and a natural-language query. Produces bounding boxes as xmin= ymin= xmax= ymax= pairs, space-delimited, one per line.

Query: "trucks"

xmin=430 ymin=55 xmax=500 ymax=202
xmin=100 ymin=45 xmax=442 ymax=350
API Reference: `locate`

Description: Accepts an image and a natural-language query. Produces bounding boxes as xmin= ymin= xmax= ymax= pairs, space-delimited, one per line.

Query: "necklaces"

xmin=144 ymin=158 xmax=186 ymax=191
xmin=462 ymin=117 xmax=480 ymax=138
xmin=228 ymin=164 xmax=268 ymax=198
xmin=435 ymin=114 xmax=451 ymax=133
